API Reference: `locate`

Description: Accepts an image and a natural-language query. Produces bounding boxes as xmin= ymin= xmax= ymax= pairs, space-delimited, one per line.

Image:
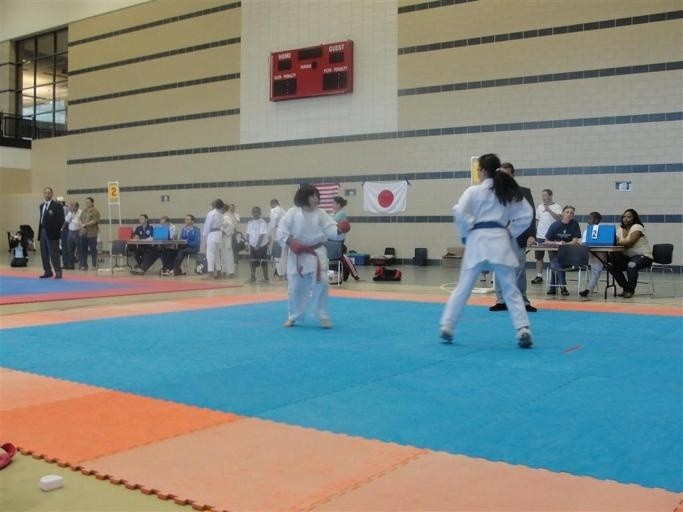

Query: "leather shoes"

xmin=40 ymin=272 xmax=53 ymax=278
xmin=55 ymin=275 xmax=62 ymax=280
xmin=488 ymin=303 xmax=506 ymax=312
xmin=526 ymin=305 xmax=537 ymax=312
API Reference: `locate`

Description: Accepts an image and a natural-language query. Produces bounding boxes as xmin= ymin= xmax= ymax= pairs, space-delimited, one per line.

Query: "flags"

xmin=363 ymin=181 xmax=407 ymax=214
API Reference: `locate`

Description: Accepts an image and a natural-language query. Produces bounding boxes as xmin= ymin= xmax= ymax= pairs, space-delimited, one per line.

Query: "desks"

xmin=125 ymin=240 xmax=186 ymax=276
xmin=524 ymin=244 xmax=624 ymax=298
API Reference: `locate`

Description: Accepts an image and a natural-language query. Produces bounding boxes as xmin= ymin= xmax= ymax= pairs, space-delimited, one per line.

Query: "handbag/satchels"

xmin=371 ymin=267 xmax=402 ymax=282
xmin=232 ymin=231 xmax=247 ymax=252
xmin=194 ymin=258 xmax=209 ymax=275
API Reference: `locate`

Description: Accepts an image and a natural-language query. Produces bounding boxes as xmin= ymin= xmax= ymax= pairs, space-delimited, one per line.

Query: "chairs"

xmin=186 ymin=241 xmax=240 ymax=280
xmin=260 ymin=241 xmax=281 ymax=288
xmin=110 ymin=240 xmax=131 ymax=274
xmin=328 ymin=240 xmax=344 ymax=286
xmin=544 ymin=255 xmax=613 ymax=298
xmin=636 ymin=244 xmax=675 ymax=295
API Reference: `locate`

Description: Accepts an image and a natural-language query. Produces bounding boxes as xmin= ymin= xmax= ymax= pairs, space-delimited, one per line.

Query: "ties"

xmin=41 ymin=202 xmax=49 ymax=224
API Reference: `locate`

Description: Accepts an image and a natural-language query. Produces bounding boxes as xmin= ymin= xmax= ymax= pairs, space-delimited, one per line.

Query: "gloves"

xmin=287 ymin=237 xmax=305 ymax=256
xmin=334 ymin=219 xmax=351 ymax=235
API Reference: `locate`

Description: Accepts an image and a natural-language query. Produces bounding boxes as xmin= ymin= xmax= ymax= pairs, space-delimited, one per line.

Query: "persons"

xmin=128 ymin=214 xmax=200 ymax=276
xmin=55 ymin=196 xmax=100 ymax=269
xmin=439 ymin=153 xmax=533 ymax=348
xmin=275 ymin=184 xmax=350 ymax=329
xmin=490 ymin=162 xmax=654 ymax=312
xmin=204 ymin=197 xmax=350 ymax=283
xmin=38 ymin=189 xmax=65 ymax=278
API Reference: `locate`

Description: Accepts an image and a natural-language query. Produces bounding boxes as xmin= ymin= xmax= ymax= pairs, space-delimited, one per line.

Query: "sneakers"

xmin=258 ymin=278 xmax=269 ymax=285
xmin=623 ymin=292 xmax=632 ymax=298
xmin=129 ymin=267 xmax=145 ymax=275
xmin=560 ymin=289 xmax=570 ymax=296
xmin=530 ymin=276 xmax=543 ymax=284
xmin=439 ymin=327 xmax=452 ymax=341
xmin=515 ymin=329 xmax=533 ymax=349
xmin=578 ymin=289 xmax=590 ymax=297
xmin=546 ymin=290 xmax=557 ymax=295
xmin=160 ymin=270 xmax=169 ymax=276
xmin=619 ymin=291 xmax=624 ymax=296
xmin=243 ymin=277 xmax=257 ymax=284
